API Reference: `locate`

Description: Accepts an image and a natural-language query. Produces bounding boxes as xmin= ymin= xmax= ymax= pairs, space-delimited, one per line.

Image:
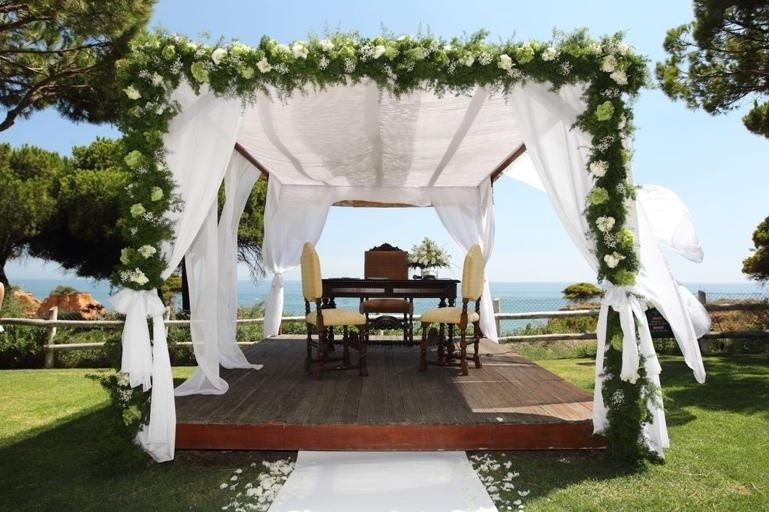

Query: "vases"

xmin=422 ymin=265 xmax=439 ymax=281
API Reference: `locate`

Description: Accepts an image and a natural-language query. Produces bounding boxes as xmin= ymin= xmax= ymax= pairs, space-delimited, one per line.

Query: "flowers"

xmin=405 ymin=237 xmax=455 ymax=271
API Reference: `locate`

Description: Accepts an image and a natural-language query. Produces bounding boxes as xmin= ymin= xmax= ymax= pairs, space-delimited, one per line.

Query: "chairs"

xmin=301 ymin=241 xmax=413 ymax=380
xmin=421 ymin=243 xmax=485 ymax=379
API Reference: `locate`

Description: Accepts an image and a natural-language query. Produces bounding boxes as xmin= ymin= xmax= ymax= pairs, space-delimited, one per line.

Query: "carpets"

xmin=264 ymin=447 xmax=498 ymax=512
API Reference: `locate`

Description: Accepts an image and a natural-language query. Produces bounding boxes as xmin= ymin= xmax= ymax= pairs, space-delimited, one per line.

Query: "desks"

xmin=322 ymin=278 xmax=461 ymax=368
